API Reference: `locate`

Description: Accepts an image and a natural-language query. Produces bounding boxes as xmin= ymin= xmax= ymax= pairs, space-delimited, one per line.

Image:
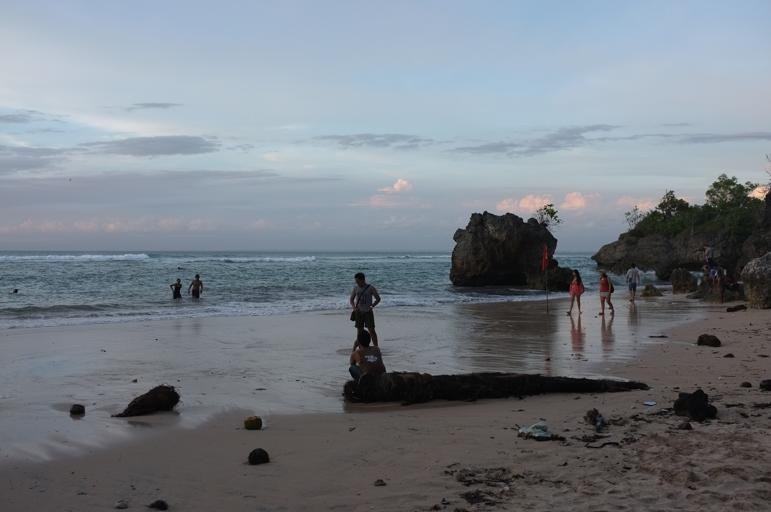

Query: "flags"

xmin=542 ymin=240 xmax=552 ymax=273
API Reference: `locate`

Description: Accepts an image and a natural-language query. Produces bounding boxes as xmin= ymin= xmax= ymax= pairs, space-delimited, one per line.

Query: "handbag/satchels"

xmin=628 ymin=277 xmax=632 ymax=282
xmin=350 ymin=310 xmax=361 ymax=321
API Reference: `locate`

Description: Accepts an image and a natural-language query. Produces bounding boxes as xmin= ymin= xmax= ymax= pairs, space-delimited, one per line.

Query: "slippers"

xmin=608 ymin=306 xmax=613 ymax=309
xmin=598 ymin=312 xmax=603 ymax=315
xmin=578 ymin=312 xmax=582 ymax=314
xmin=566 ymin=312 xmax=571 ymax=314
xmin=629 ymin=299 xmax=633 ymax=302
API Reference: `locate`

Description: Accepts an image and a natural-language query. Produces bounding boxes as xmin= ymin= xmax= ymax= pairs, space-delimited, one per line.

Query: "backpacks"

xmin=606 ymin=276 xmax=614 ymax=293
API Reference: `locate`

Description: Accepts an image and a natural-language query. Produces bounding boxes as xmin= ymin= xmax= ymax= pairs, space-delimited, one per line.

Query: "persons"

xmin=601 ymin=316 xmax=615 ymax=351
xmin=627 ymin=303 xmax=642 ymax=338
xmin=188 ymin=275 xmax=204 ymax=299
xmin=170 ymin=278 xmax=183 ymax=299
xmin=349 ymin=329 xmax=384 ymax=382
xmin=570 ymin=314 xmax=588 ymax=354
xmin=566 ymin=268 xmax=587 ymax=315
xmin=625 ymin=263 xmax=642 ymax=303
xmin=350 ymin=272 xmax=381 ymax=347
xmin=597 ymin=268 xmax=617 ymax=315
